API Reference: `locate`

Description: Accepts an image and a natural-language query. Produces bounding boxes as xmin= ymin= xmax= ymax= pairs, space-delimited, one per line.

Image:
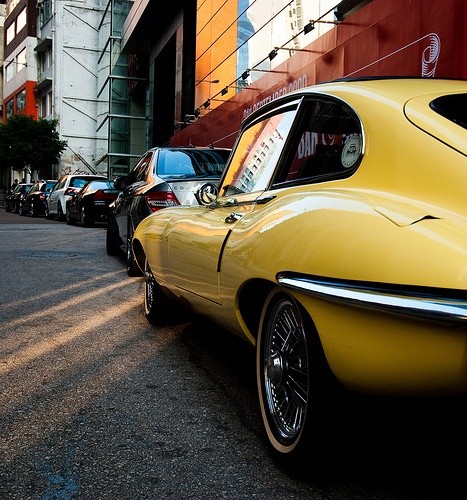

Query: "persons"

xmin=11 ymin=179 xmax=19 ymax=191
xmin=19 ymin=178 xmax=26 ymax=184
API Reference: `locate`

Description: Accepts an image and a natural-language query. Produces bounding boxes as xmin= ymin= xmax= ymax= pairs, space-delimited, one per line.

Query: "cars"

xmin=5 ymin=174 xmax=109 ymax=224
xmin=131 ymin=76 xmax=467 ymax=464
xmin=66 ymin=180 xmax=122 ymax=226
xmin=106 ymin=146 xmax=232 ymax=276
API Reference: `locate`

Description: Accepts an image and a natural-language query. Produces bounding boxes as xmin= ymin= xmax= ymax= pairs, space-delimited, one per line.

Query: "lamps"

xmin=203 ymin=98 xmax=245 ymax=108
xmin=220 ymin=85 xmax=262 ymax=96
xmin=268 ymin=46 xmax=324 ymax=62
xmin=194 ymin=107 xmax=214 ymax=119
xmin=241 ymin=67 xmax=289 ymax=80
xmin=304 ymin=18 xmax=340 ymax=35
xmin=175 ymin=120 xmax=193 ymax=129
xmin=184 ymin=114 xmax=204 ymax=123
